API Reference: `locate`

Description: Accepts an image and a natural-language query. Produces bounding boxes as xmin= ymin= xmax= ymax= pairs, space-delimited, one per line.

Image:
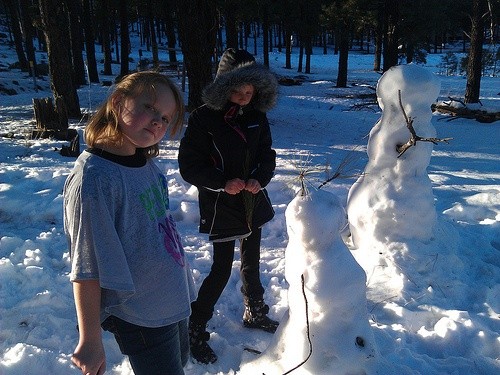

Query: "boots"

xmin=188 ymin=321 xmax=218 ymax=364
xmin=243 ymin=299 xmax=280 ymax=333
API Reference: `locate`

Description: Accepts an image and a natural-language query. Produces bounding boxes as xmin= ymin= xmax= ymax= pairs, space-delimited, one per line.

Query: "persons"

xmin=62 ymin=69 xmax=198 ymax=375
xmin=178 ymin=46 xmax=280 ymax=366
